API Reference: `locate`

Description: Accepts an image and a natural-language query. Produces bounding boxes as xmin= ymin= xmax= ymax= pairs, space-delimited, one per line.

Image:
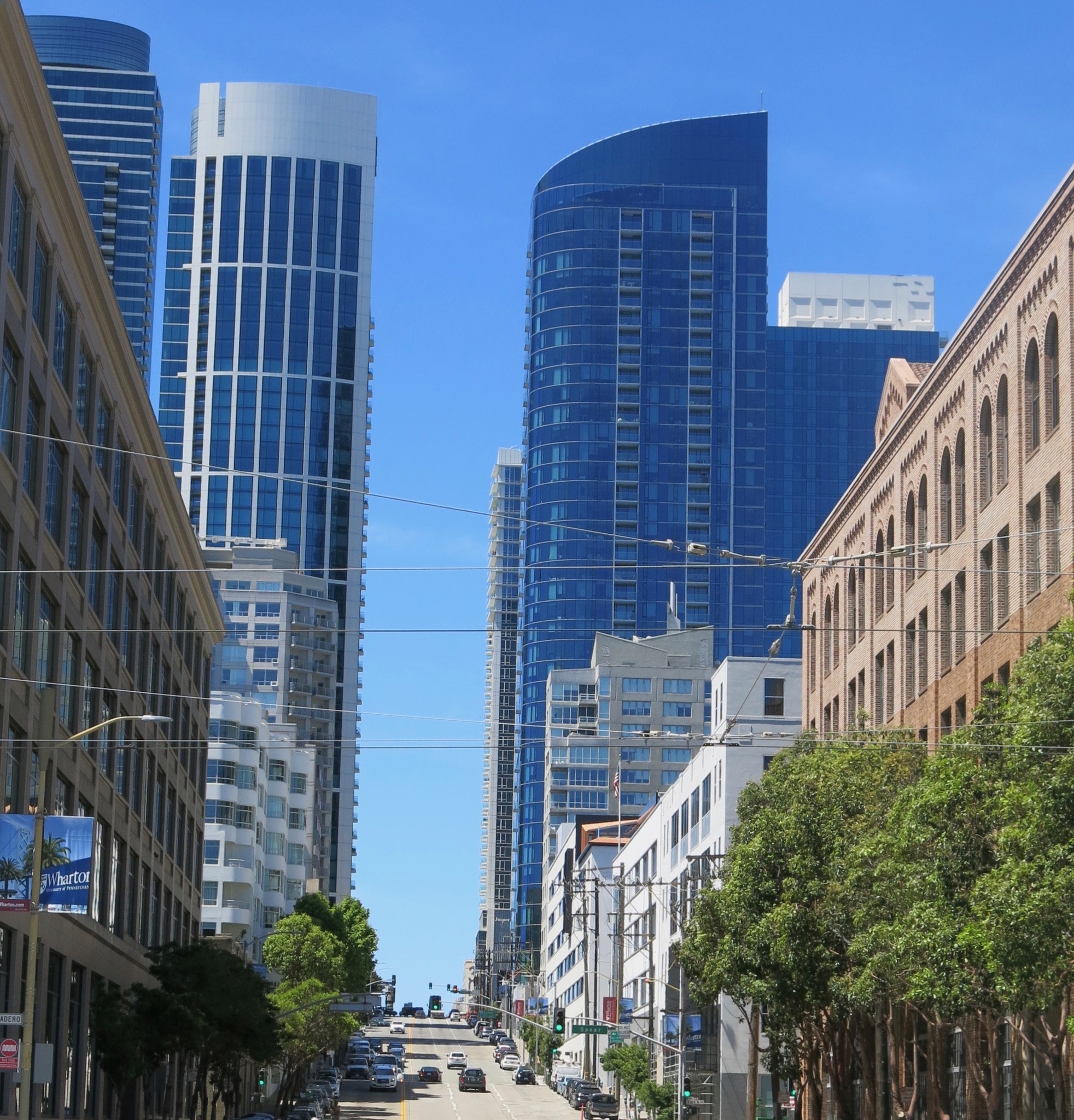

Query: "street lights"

xmin=519 ymin=975 xmax=538 ymax=1072
xmin=17 ymin=714 xmax=177 ymax=1120
xmin=491 ymin=973 xmax=511 ymax=1038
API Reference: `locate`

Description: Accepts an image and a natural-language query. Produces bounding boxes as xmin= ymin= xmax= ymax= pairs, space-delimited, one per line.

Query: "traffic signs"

xmin=610 ymin=1031 xmax=624 ymax=1043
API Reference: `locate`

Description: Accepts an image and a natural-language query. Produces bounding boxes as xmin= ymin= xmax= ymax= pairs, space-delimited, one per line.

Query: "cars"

xmin=449 ymin=1008 xmax=521 ymax=1064
xmin=390 ymin=1021 xmax=406 ymax=1034
xmin=446 ymin=1051 xmax=468 ymax=1069
xmin=556 ymin=1074 xmax=602 ymax=1110
xmin=500 ymin=1055 xmax=521 ymax=1071
xmin=367 ymin=1017 xmax=389 ymax=1027
xmin=511 ymin=1065 xmax=536 ymax=1085
xmin=457 ymin=1067 xmax=486 ymax=1092
xmin=373 ymin=1006 xmax=426 ymax=1020
xmin=418 ymin=1067 xmax=442 ymax=1083
xmin=344 ymin=1031 xmax=407 ymax=1093
xmin=235 ymin=1066 xmax=343 ymax=1120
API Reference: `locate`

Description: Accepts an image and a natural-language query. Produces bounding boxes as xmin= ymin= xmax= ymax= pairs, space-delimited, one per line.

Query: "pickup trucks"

xmin=582 ymin=1093 xmax=619 ymax=1120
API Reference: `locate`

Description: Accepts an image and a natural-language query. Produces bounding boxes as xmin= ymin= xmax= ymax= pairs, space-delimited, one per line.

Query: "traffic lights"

xmin=684 ymin=1075 xmax=691 ymax=1096
xmin=447 ymin=984 xmax=451 ymax=991
xmin=430 ymin=996 xmax=442 ymax=1011
xmin=552 ymin=1040 xmax=557 ymax=1052
xmin=429 ymin=983 xmax=432 ymax=989
xmin=453 ymin=985 xmax=458 ymax=993
xmin=258 ymin=1067 xmax=265 ymax=1087
xmin=552 ymin=1008 xmax=566 ymax=1034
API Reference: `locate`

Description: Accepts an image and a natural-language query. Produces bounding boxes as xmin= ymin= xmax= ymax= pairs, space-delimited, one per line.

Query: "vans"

xmin=549 ymin=1065 xmax=582 ymax=1092
